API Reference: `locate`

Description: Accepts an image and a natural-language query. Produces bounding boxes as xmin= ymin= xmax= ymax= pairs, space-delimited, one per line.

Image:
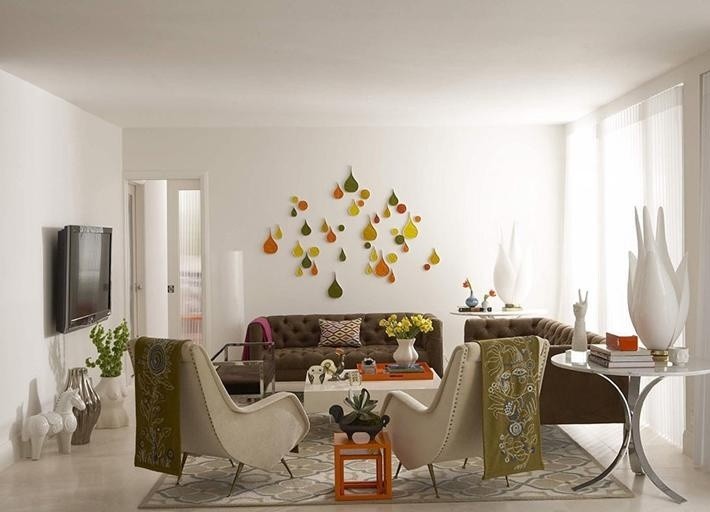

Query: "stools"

xmin=334 ymin=433 xmax=394 ymax=500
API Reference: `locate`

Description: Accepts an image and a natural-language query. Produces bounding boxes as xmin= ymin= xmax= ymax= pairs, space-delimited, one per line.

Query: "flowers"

xmin=484 ymin=290 xmax=496 ymax=301
xmin=379 ymin=313 xmax=435 ymax=338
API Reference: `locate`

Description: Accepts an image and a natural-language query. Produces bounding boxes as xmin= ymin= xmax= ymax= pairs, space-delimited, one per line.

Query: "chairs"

xmin=210 ymin=342 xmax=276 ymax=400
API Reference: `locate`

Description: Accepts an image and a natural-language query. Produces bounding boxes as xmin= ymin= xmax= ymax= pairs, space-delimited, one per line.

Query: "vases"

xmin=394 ymin=338 xmax=419 ymax=369
xmin=466 ymin=290 xmax=479 ymax=307
xmin=481 ymin=302 xmax=488 ymax=309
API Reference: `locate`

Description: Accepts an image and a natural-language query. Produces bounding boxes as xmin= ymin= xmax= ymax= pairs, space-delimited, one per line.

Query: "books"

xmin=589 ymin=351 xmax=653 ymax=362
xmin=502 ymin=306 xmax=522 ymax=312
xmin=590 ymin=344 xmax=650 ymax=356
xmin=589 ymin=354 xmax=656 ymax=369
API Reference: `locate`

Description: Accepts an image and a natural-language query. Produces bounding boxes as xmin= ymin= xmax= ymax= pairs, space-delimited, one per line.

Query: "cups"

xmin=349 ymin=371 xmax=362 ymax=386
xmin=668 ymin=346 xmax=690 ymax=366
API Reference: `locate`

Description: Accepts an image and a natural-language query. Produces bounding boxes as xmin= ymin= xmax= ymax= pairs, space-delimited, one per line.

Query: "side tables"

xmin=450 ymin=311 xmax=527 ymax=319
xmin=552 ymin=352 xmax=710 ymax=504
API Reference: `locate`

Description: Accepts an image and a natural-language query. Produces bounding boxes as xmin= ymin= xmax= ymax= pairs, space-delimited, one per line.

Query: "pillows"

xmin=319 ymin=318 xmax=361 ymax=348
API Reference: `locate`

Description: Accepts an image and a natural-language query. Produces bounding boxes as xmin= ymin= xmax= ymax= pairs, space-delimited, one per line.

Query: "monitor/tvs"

xmin=56 ymin=225 xmax=114 ymax=334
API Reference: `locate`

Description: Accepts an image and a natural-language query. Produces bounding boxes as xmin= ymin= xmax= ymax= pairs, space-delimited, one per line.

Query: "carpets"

xmin=137 ymin=393 xmax=615 ymax=509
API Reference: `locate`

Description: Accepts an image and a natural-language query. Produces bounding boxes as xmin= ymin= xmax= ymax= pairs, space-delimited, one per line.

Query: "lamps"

xmin=626 ymin=205 xmax=692 ymax=369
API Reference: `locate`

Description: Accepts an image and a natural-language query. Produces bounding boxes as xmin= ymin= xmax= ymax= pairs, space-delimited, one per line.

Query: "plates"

xmin=356 ymin=361 xmax=434 ymax=381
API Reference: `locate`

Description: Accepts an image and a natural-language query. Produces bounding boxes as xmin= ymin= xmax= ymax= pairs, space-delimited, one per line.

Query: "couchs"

xmin=128 ymin=337 xmax=311 ymax=497
xmin=248 ymin=312 xmax=443 ymax=380
xmin=380 ymin=336 xmax=551 ymax=499
xmin=464 ymin=318 xmax=630 ymax=426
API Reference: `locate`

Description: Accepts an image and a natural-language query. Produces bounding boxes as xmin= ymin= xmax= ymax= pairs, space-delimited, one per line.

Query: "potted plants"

xmin=86 ymin=318 xmax=129 ymax=427
